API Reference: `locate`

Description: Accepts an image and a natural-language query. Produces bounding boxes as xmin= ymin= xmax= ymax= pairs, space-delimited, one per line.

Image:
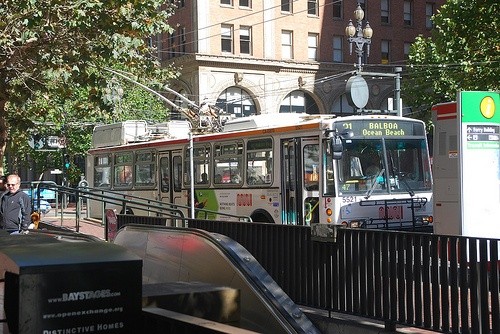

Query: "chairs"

xmin=350 ymin=157 xmax=364 ymax=176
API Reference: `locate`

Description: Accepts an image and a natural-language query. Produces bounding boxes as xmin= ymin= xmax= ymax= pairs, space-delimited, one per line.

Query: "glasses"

xmin=7 ymin=182 xmax=19 ymax=186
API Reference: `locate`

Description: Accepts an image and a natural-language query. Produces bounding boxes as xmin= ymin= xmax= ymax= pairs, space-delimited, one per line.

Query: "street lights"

xmin=345 ymin=2 xmax=374 ymax=75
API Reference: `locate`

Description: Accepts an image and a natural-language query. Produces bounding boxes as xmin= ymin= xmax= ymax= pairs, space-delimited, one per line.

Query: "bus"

xmin=20 ymin=181 xmax=59 ymax=200
xmin=83 ymin=64 xmax=436 ymax=234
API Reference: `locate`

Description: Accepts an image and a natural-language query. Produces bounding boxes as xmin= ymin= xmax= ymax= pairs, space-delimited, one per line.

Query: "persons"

xmin=197 ymin=173 xmax=209 ymax=184
xmin=215 ymin=174 xmax=223 ymax=183
xmin=0 ymin=174 xmax=31 ymax=234
xmin=228 ymin=174 xmax=239 ymax=183
xmin=366 ymin=155 xmax=384 ymax=176
xmin=188 ymin=190 xmax=207 ymax=218
xmin=250 ymin=170 xmax=257 ymax=180
